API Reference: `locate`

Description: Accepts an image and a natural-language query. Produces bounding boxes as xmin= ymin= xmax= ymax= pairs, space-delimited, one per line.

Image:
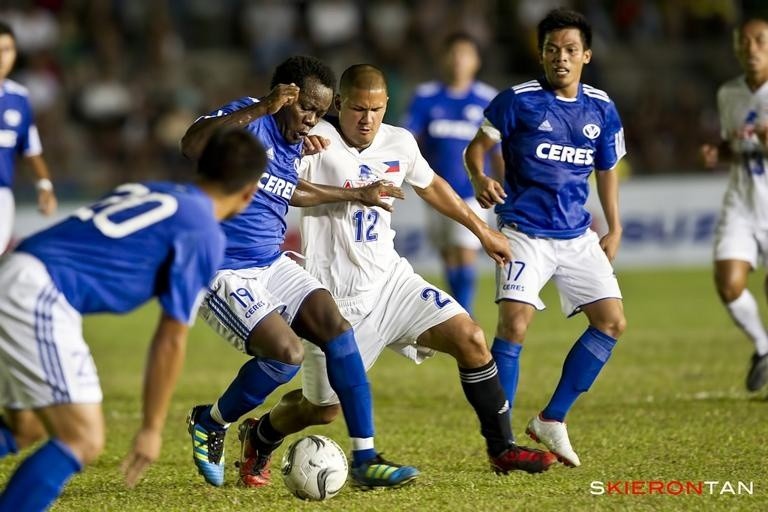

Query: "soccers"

xmin=280 ymin=434 xmax=349 ymax=502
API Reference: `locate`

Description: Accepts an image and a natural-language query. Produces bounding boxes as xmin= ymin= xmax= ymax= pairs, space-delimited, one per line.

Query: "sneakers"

xmin=747 ymin=350 xmax=768 ymax=390
xmin=526 ymin=412 xmax=580 ymax=464
xmin=187 ymin=404 xmax=231 ymax=486
xmin=239 ymin=417 xmax=273 ymax=486
xmin=492 ymin=441 xmax=555 ymax=475
xmin=350 ymin=452 xmax=419 ymax=490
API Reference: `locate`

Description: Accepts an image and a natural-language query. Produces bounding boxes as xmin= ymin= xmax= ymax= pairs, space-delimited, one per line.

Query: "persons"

xmin=0 ymin=125 xmax=267 ymax=512
xmin=0 ymin=23 xmax=57 ymax=261
xmin=237 ymin=64 xmax=557 ymax=489
xmin=180 ymin=52 xmax=419 ymax=492
xmin=462 ymin=8 xmax=628 ymax=468
xmin=406 ymin=31 xmax=506 ymax=317
xmin=701 ymin=18 xmax=768 ymax=404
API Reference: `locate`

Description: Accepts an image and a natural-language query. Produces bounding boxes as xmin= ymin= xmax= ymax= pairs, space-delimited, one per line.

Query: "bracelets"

xmin=34 ymin=178 xmax=53 ymax=191
